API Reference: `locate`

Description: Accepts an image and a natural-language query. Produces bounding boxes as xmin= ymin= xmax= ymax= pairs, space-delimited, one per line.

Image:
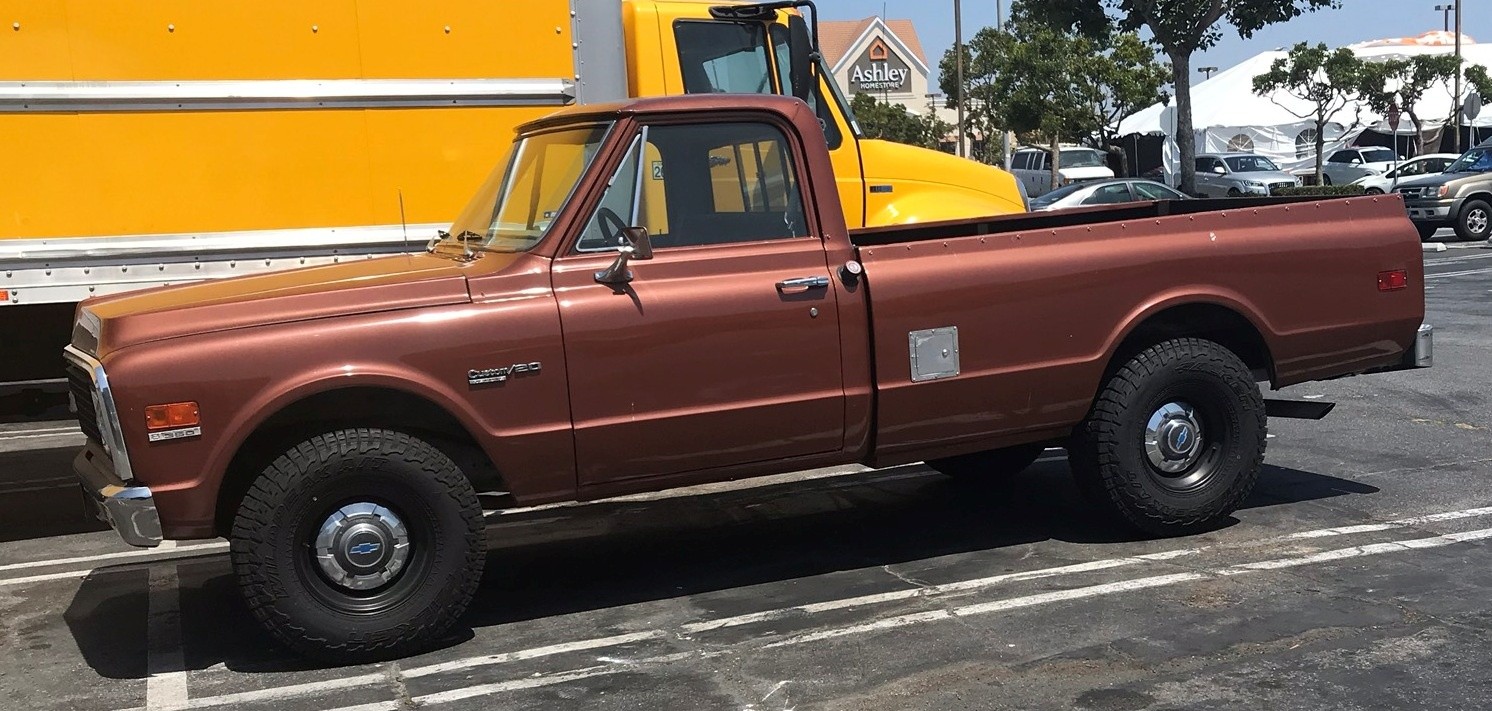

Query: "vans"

xmin=1007 ymin=147 xmax=1115 ymax=197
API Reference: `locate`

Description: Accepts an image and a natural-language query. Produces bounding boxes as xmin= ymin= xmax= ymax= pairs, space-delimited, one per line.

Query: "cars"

xmin=1322 ymin=146 xmax=1405 ymax=186
xmin=1355 ymin=153 xmax=1480 ymax=195
xmin=1028 ymin=177 xmax=1193 ymax=214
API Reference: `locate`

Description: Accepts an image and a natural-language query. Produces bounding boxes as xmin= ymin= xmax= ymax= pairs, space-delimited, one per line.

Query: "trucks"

xmin=2 ymin=1 xmax=1032 ymax=311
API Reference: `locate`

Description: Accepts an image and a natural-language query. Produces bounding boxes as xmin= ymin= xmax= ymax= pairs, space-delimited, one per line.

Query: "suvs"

xmin=1391 ymin=137 xmax=1492 ymax=241
xmin=1195 ymin=152 xmax=1302 ymax=198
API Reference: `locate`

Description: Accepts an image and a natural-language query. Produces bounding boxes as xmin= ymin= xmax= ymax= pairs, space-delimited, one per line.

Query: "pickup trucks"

xmin=59 ymin=90 xmax=1436 ymax=665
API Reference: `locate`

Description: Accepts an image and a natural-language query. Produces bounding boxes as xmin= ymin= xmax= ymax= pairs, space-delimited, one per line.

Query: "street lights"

xmin=924 ymin=93 xmax=941 ymax=151
xmin=1198 ymin=67 xmax=1219 ymax=80
xmin=1435 ymin=5 xmax=1455 ymax=32
